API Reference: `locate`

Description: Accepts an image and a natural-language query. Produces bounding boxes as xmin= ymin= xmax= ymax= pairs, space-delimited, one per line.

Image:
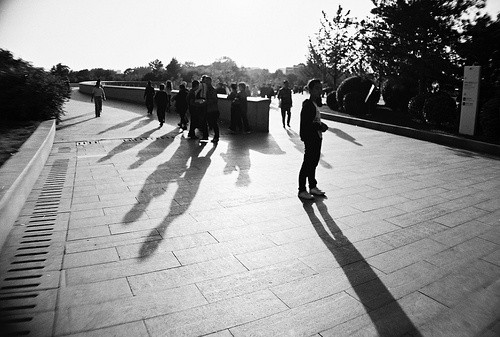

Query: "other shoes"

xmin=310 ymin=187 xmax=325 ymax=195
xmin=287 ymin=124 xmax=290 ymax=127
xmin=182 ymin=126 xmax=188 ymax=130
xmin=211 ymin=139 xmax=219 ymax=142
xmin=298 ymin=191 xmax=314 ymax=199
xmin=178 ymin=123 xmax=182 ymax=127
xmin=201 ymin=133 xmax=209 ymax=140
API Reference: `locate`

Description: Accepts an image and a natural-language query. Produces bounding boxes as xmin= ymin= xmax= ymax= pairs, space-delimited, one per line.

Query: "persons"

xmin=188 ymin=74 xmax=221 ymax=143
xmin=171 ymin=85 xmax=190 ymax=130
xmin=226 ymin=83 xmax=249 ymax=131
xmin=245 ymin=83 xmax=276 ymax=106
xmin=277 ymin=80 xmax=292 ymax=128
xmin=153 ymin=83 xmax=168 ymax=122
xmin=144 ymin=81 xmax=155 ymax=115
xmin=90 ymin=79 xmax=106 ymax=118
xmin=298 ymin=79 xmax=327 ymax=201
